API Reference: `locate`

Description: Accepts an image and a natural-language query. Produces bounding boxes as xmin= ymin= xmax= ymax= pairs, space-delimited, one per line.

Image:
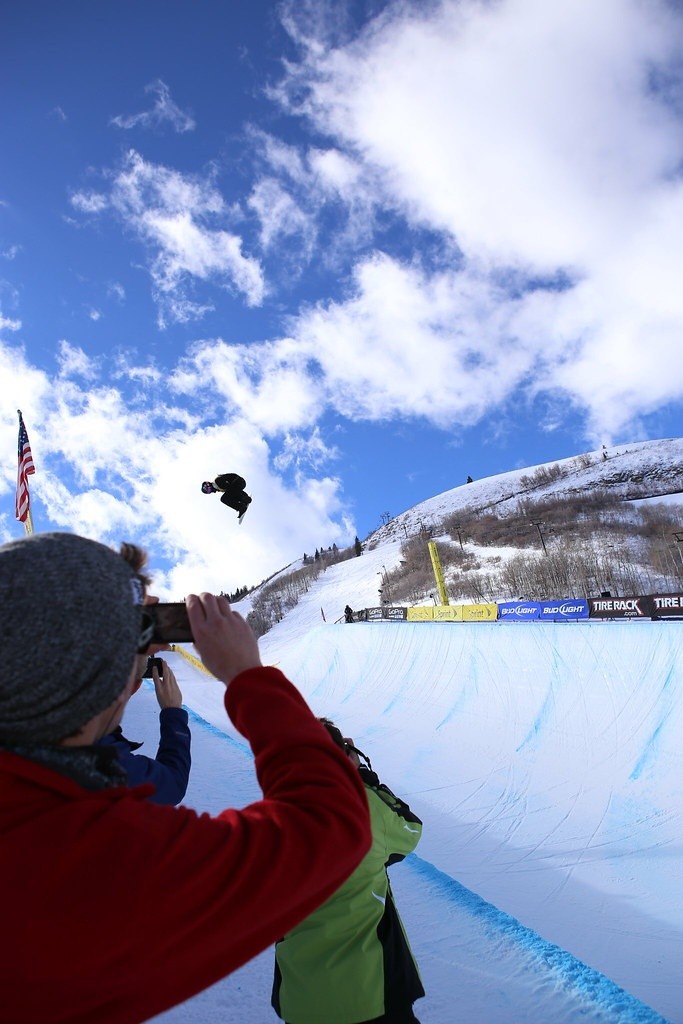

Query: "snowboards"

xmin=239 ymin=494 xmax=254 ymax=525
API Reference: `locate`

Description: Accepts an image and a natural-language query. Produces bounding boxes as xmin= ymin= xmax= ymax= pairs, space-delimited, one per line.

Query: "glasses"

xmin=207 ymin=486 xmax=211 ymax=494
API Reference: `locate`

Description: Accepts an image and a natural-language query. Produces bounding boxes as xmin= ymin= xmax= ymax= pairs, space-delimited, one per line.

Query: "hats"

xmin=202 ymin=482 xmax=212 ymax=494
xmin=0 ymin=532 xmax=143 ymax=748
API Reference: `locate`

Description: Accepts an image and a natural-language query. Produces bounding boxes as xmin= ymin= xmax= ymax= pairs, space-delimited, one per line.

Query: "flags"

xmin=15 ymin=412 xmax=35 ymax=523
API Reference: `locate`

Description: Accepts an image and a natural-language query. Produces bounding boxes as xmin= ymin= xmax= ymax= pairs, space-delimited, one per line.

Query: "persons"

xmin=97 ymin=661 xmax=192 ymax=805
xmin=201 ymin=473 xmax=252 ymax=518
xmin=0 ymin=531 xmax=374 ymax=1024
xmin=270 ymin=718 xmax=426 ymax=1024
xmin=345 ymin=605 xmax=355 ymax=623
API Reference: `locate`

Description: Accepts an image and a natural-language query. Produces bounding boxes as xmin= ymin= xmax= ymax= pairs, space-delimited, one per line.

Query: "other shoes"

xmin=238 ymin=506 xmax=247 ymax=518
xmin=240 ymin=497 xmax=251 ymax=504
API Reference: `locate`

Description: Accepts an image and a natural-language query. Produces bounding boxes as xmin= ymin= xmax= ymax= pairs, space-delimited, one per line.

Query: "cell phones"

xmin=145 ymin=602 xmax=194 ymax=643
xmin=141 ymin=658 xmax=163 ymax=678
